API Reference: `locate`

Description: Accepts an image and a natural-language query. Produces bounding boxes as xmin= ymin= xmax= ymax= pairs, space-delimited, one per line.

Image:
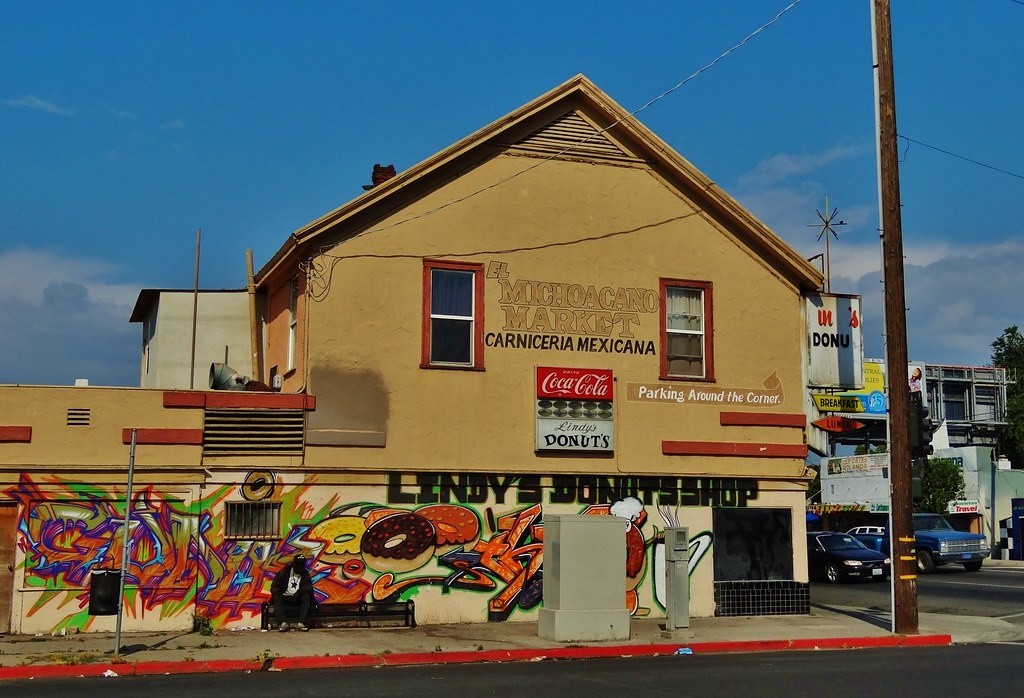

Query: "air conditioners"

xmin=273 ymin=375 xmax=281 ymax=389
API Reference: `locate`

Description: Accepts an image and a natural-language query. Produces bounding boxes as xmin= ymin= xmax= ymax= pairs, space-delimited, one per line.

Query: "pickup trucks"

xmin=852 ymin=511 xmax=992 ymax=575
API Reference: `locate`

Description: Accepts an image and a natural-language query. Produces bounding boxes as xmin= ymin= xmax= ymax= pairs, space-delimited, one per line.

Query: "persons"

xmin=270 ymin=554 xmax=314 ymax=632
xmin=908 ymin=368 xmax=923 ymax=392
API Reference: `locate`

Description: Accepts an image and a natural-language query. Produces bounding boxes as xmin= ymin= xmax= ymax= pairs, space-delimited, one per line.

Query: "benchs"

xmin=261 ymin=599 xmax=416 ymax=629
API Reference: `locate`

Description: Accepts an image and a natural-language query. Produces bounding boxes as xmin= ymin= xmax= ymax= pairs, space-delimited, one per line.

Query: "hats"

xmin=293 ymin=554 xmax=305 ymax=574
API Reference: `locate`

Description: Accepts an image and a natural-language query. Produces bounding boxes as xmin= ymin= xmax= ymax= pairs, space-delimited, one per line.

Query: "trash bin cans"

xmin=87 ymin=567 xmax=121 ymax=616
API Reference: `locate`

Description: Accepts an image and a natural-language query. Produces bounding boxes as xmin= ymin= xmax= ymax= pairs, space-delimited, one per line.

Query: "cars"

xmin=806 ymin=531 xmax=891 ymax=585
xmin=845 ymin=526 xmax=885 ymax=536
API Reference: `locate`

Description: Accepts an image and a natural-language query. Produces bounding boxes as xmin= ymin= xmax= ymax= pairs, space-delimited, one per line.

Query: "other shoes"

xmin=279 ymin=622 xmax=290 ymax=632
xmin=297 ymin=623 xmax=308 ymax=631
